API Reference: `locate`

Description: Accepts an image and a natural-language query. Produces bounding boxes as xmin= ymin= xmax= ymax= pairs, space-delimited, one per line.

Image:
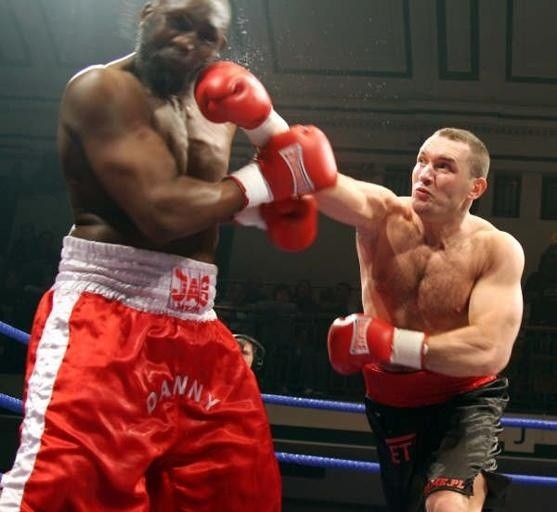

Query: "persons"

xmin=215 ymin=276 xmax=364 ymax=402
xmin=227 ymin=322 xmax=264 ymax=373
xmin=503 ymin=244 xmax=556 ymax=414
xmin=193 ymin=60 xmax=526 ymax=512
xmin=2 ymin=242 xmax=51 ymax=375
xmin=0 ymin=0 xmax=337 ymax=512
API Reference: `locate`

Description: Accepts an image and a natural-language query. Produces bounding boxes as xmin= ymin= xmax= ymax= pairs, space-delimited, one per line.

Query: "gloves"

xmin=328 ymin=313 xmax=429 ymax=375
xmin=194 ymin=61 xmax=290 ymax=148
xmin=233 ymin=194 xmax=318 ymax=252
xmin=222 ymin=124 xmax=338 ymax=211
